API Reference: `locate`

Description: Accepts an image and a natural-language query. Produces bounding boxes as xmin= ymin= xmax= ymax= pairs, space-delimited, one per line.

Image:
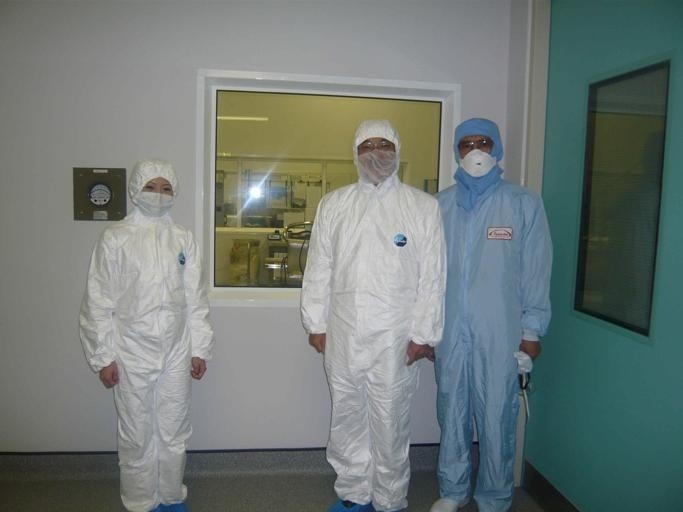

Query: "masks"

xmin=459 ymin=149 xmax=497 ymax=181
xmin=133 ymin=191 xmax=174 ymax=217
xmin=357 ymin=150 xmax=398 ymax=186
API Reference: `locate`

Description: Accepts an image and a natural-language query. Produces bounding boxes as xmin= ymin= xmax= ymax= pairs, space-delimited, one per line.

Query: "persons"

xmin=419 ymin=117 xmax=552 ymax=512
xmin=78 ymin=159 xmax=213 ymax=510
xmin=297 ymin=120 xmax=445 ymax=512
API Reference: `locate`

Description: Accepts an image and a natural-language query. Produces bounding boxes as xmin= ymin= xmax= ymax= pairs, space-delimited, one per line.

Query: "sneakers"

xmin=328 ymin=498 xmax=373 ymax=512
xmin=428 ymin=496 xmax=464 ymax=512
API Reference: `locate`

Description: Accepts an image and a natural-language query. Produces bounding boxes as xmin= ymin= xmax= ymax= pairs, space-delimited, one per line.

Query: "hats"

xmin=452 ymin=118 xmax=502 ymax=163
xmin=354 ymin=118 xmax=401 ymax=156
xmin=128 ymin=161 xmax=179 ymax=202
